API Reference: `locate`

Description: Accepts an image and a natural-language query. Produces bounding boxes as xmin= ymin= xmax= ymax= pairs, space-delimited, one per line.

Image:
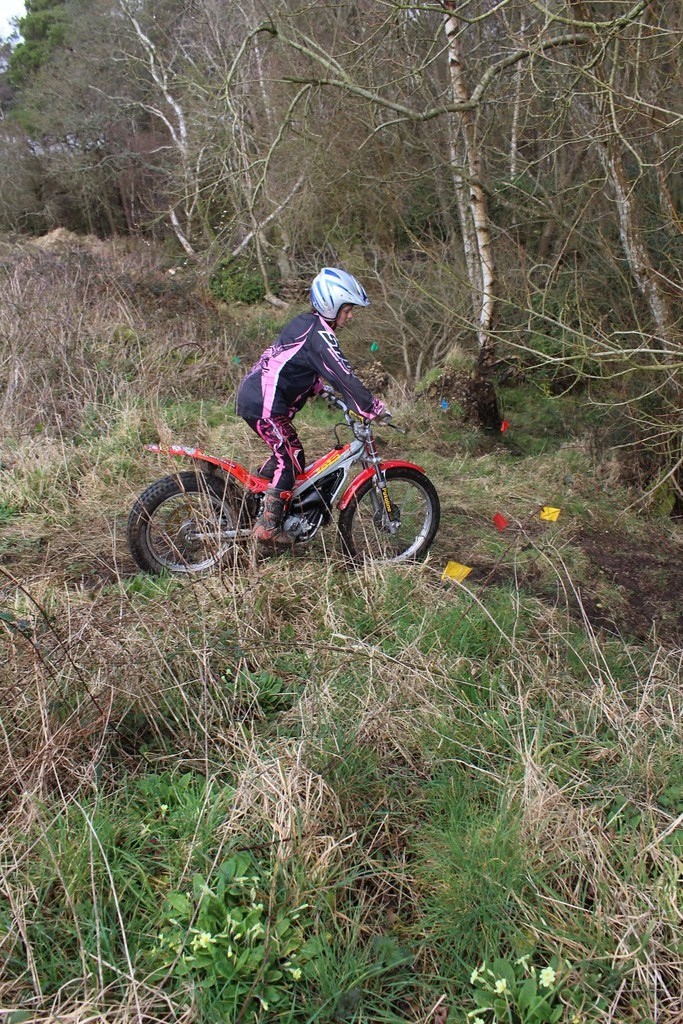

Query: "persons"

xmin=235 ymin=267 xmax=391 ymax=548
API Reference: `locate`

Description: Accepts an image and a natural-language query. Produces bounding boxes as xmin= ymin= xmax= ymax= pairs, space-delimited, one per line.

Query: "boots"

xmin=253 ymin=487 xmax=295 ymax=547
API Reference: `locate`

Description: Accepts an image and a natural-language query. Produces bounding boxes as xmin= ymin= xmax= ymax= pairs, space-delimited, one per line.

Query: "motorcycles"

xmin=126 ymin=394 xmax=441 ymax=577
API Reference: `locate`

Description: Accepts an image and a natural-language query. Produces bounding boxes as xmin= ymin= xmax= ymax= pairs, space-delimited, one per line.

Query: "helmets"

xmin=310 ymin=267 xmax=371 ymax=322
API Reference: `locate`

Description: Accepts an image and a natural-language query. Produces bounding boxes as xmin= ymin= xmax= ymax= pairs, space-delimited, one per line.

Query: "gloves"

xmin=371 ymin=398 xmax=393 ymax=428
xmin=319 ymin=385 xmax=336 ymax=405
